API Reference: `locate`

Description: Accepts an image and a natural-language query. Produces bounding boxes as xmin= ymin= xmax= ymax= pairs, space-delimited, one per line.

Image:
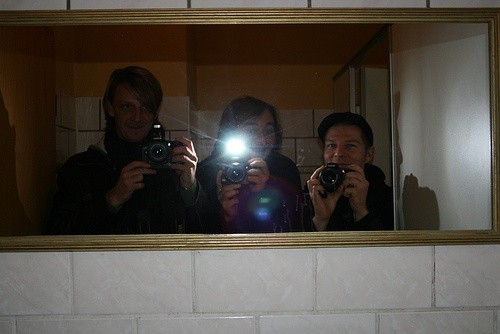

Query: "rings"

xmin=219 ymin=187 xmax=223 ymax=192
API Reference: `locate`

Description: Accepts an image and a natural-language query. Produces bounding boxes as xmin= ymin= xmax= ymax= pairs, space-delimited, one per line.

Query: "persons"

xmin=301 ymin=112 xmax=394 ymax=232
xmin=44 ymin=65 xmax=211 ymax=236
xmin=186 ymin=96 xmax=306 ymax=235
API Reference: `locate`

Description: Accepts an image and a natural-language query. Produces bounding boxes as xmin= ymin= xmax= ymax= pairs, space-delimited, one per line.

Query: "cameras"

xmin=220 ymin=156 xmax=258 ymax=185
xmin=142 ymin=140 xmax=186 ymax=169
xmin=315 ymin=163 xmax=357 ymax=197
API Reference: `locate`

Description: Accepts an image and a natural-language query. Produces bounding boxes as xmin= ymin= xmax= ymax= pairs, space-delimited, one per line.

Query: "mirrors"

xmin=0 ymin=7 xmax=500 ymax=254
